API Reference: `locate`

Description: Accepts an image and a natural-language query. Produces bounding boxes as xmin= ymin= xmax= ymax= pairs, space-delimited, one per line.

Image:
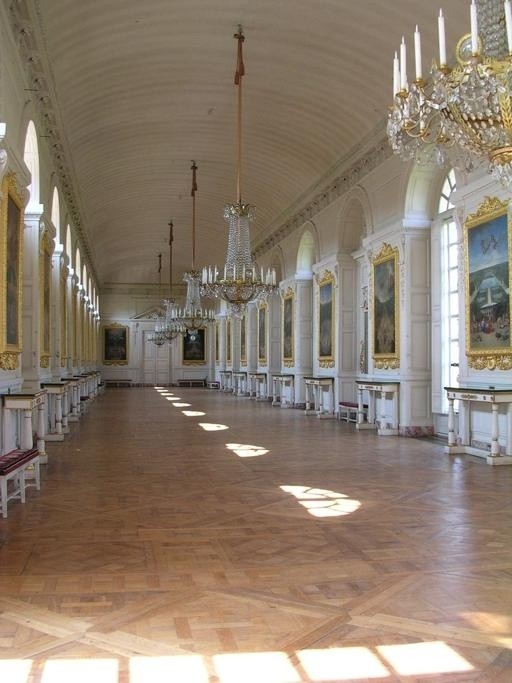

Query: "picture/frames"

xmin=316 ymin=270 xmax=335 ymax=367
xmin=216 ymin=288 xmax=297 ymax=367
xmin=368 ymin=244 xmax=400 ymax=370
xmin=462 ymin=197 xmax=512 ymax=373
xmin=41 ymin=230 xmax=53 ymax=366
xmin=180 ymin=326 xmax=208 ymax=367
xmin=0 ymin=168 xmax=24 ymax=371
xmin=103 ymin=322 xmax=131 ymax=367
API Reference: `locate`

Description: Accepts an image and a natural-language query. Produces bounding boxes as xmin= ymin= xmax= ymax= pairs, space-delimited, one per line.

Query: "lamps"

xmin=387 ymin=0 xmax=512 ymax=196
xmin=172 ymin=160 xmax=217 ymax=341
xmin=155 ymin=222 xmax=186 ymax=345
xmin=145 ymin=253 xmax=168 ymax=346
xmin=201 ymin=29 xmax=277 ymax=319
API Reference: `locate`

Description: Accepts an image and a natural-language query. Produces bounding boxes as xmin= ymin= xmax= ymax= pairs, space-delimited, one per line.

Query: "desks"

xmin=0 ymin=370 xmax=103 ymax=478
xmin=217 ymin=371 xmax=512 ymax=467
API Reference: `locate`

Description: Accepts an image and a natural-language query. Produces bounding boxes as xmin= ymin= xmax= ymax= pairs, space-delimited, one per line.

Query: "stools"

xmin=0 ymin=448 xmax=40 ymax=519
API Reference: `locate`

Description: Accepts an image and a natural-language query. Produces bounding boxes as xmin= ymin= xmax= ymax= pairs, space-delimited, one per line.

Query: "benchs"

xmin=104 ymin=379 xmax=133 ymax=387
xmin=176 ymin=379 xmax=205 ymax=388
